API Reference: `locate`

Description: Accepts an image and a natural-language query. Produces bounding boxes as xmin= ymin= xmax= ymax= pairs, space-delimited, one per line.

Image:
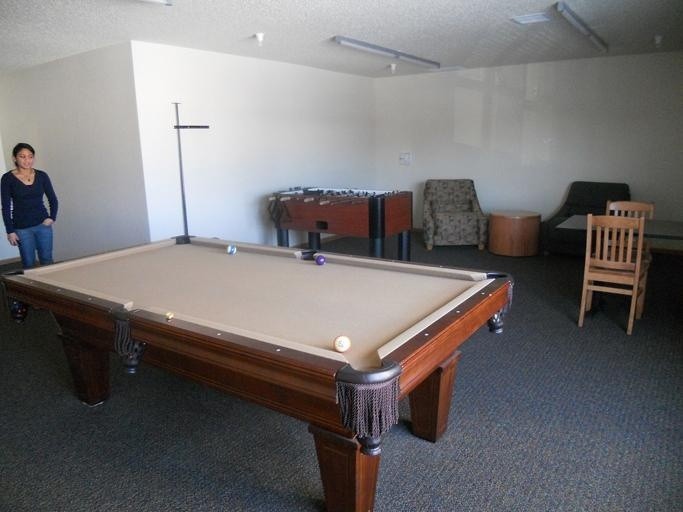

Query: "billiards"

xmin=316 ymin=255 xmax=326 ymax=265
xmin=227 ymin=245 xmax=236 ymax=254
xmin=334 ymin=336 xmax=350 ymax=352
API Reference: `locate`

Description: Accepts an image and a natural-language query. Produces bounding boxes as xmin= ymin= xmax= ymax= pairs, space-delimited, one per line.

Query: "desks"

xmin=553 ymin=212 xmax=682 ymax=316
xmin=0 ymin=231 xmax=518 ymax=512
xmin=488 ymin=209 xmax=541 ymax=257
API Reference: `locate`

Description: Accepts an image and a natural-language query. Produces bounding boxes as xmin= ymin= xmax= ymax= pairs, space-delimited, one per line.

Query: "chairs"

xmin=577 ymin=214 xmax=647 ymax=335
xmin=602 ymin=198 xmax=656 ymax=276
xmin=544 ymin=179 xmax=629 ymax=262
xmin=421 ymin=176 xmax=489 ymax=250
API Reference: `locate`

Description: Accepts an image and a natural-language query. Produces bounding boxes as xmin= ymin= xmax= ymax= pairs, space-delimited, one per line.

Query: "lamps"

xmin=552 ymin=1 xmax=609 ymax=55
xmin=333 ymin=35 xmax=441 ymax=70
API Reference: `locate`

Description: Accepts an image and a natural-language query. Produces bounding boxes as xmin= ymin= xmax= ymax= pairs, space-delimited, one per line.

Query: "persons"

xmin=1 ymin=142 xmax=59 ymax=311
